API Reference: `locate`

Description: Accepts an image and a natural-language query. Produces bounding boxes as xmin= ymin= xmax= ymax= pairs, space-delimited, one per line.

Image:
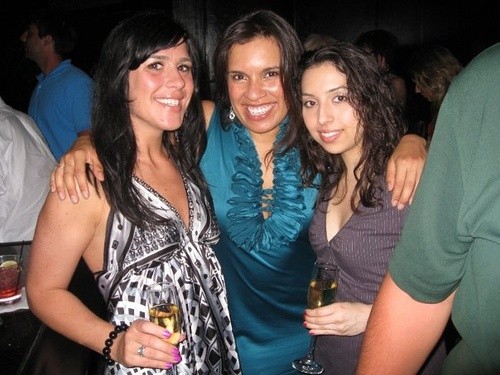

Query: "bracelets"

xmin=103 ymin=323 xmax=129 ymax=366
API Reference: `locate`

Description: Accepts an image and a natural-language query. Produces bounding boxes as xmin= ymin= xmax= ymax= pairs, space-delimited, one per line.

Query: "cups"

xmin=0 ymin=255 xmax=23 ymax=306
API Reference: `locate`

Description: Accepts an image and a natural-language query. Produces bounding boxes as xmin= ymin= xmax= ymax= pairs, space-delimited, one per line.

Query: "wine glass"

xmin=292 ymin=262 xmax=339 ymax=374
xmin=146 ymin=282 xmax=181 ymax=375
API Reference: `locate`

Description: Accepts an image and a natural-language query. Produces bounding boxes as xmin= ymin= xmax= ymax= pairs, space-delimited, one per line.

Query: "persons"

xmin=0 ymin=96 xmax=58 ymax=244
xmin=358 ymin=42 xmax=500 ymax=375
xmin=292 ymin=43 xmax=447 ymax=375
xmin=20 ymin=9 xmax=98 ymax=164
xmin=50 ymin=10 xmax=427 ymax=375
xmin=390 ymin=49 xmax=462 ymax=141
xmin=26 ymin=15 xmax=243 ymax=375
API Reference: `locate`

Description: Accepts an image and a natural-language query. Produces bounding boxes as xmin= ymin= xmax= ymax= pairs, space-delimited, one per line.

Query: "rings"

xmin=137 ymin=345 xmax=146 ymax=357
